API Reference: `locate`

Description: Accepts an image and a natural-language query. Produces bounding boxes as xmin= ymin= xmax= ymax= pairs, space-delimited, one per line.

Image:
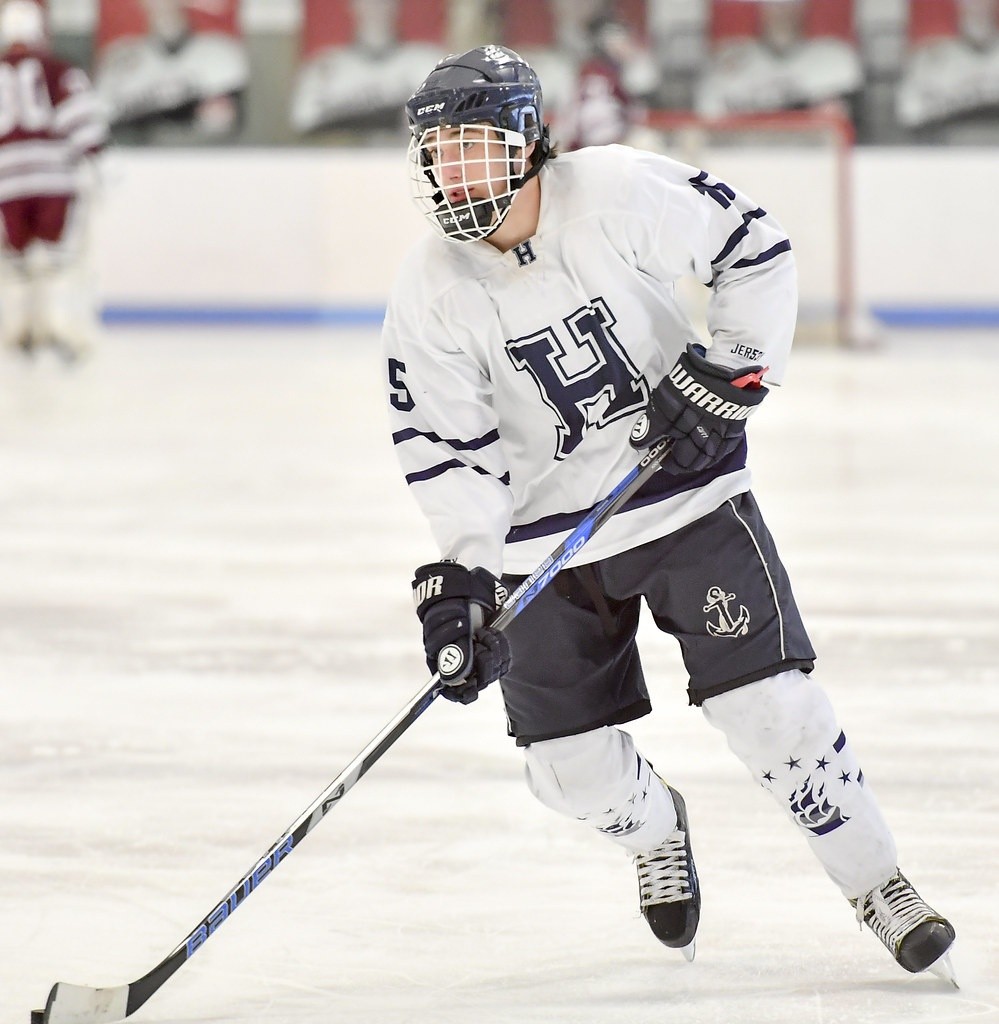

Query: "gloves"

xmin=411 ymin=562 xmax=513 ymax=705
xmin=628 ymin=342 xmax=769 ymax=476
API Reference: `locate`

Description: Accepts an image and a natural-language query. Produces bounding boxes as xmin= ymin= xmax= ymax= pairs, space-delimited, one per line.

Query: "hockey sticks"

xmin=40 ymin=434 xmax=671 ymax=1021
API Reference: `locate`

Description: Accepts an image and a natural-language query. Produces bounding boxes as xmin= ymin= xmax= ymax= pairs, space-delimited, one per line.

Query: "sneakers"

xmin=847 ymin=866 xmax=960 ymax=990
xmin=626 ymin=773 xmax=701 ymax=962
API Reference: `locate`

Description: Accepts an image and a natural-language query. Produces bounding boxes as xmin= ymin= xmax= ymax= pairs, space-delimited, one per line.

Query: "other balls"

xmin=30 ymin=1009 xmax=45 ymax=1024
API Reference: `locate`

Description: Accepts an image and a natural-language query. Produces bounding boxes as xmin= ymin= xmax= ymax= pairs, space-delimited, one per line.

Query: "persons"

xmin=1 ymin=0 xmax=101 ymax=375
xmin=290 ymin=0 xmax=998 ymax=144
xmin=89 ymin=0 xmax=248 ymax=145
xmin=379 ymin=46 xmax=961 ymax=990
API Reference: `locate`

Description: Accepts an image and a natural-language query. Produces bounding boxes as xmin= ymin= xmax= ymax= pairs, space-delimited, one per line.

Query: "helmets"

xmin=404 ymin=44 xmax=550 ymax=245
